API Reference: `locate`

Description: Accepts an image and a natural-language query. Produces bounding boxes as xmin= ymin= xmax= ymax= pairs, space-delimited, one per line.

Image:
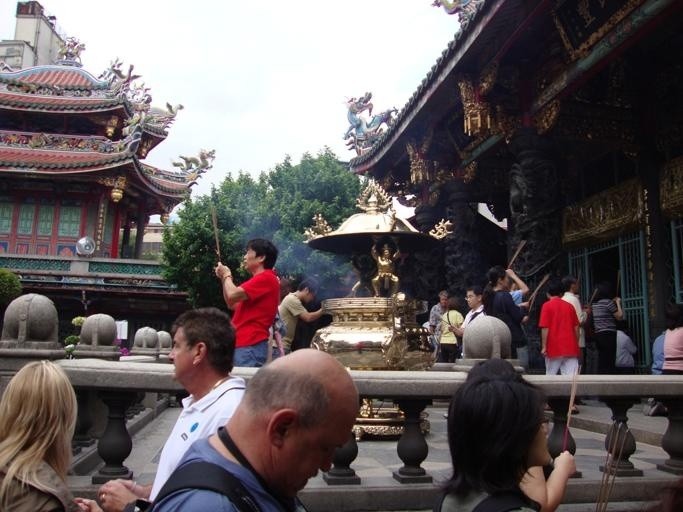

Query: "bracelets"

xmin=222 ymin=276 xmax=232 ymax=283
xmin=131 ymin=481 xmax=136 ymax=493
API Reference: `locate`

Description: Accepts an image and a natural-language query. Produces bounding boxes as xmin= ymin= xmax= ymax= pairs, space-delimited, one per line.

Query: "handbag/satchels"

xmin=502 ymin=315 xmax=526 ymax=348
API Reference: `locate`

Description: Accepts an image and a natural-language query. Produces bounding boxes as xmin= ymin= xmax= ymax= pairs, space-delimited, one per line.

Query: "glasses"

xmin=538 ymin=420 xmax=549 ymax=434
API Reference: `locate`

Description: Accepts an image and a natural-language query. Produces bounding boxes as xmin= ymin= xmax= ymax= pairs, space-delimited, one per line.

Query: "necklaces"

xmin=212 ymin=377 xmax=229 ymax=390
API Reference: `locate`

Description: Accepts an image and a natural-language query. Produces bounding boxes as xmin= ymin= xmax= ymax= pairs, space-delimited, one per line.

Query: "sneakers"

xmin=643 ymin=398 xmax=664 ymax=417
xmin=571 ymin=402 xmax=585 ymax=414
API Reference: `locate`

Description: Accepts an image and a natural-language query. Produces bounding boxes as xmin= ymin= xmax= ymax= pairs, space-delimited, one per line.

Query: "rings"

xmin=102 ymin=493 xmax=105 ymax=501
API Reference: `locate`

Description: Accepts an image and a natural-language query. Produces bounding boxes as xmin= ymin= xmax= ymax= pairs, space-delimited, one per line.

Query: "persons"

xmin=371 ymin=243 xmax=401 ymax=297
xmin=615 ymin=319 xmax=637 ymax=375
xmin=266 ymin=266 xmax=287 ymax=362
xmin=429 ymin=268 xmax=530 ymax=363
xmin=433 ymin=375 xmax=550 ymax=512
xmin=661 ymin=304 xmax=683 ymax=375
xmin=561 ymin=278 xmax=592 ymax=403
xmin=538 ymin=280 xmax=581 ymax=376
xmin=593 ymin=282 xmax=623 ymax=402
xmin=0 ymin=361 xmax=103 ymax=512
xmin=97 ymin=307 xmax=245 ymax=510
xmin=648 ymin=331 xmax=667 ymax=411
xmin=278 ymin=276 xmax=324 ymax=356
xmin=466 ymin=359 xmax=576 ymax=512
xmin=215 ymin=238 xmax=281 ymax=368
xmin=149 ymin=347 xmax=360 ymax=511
